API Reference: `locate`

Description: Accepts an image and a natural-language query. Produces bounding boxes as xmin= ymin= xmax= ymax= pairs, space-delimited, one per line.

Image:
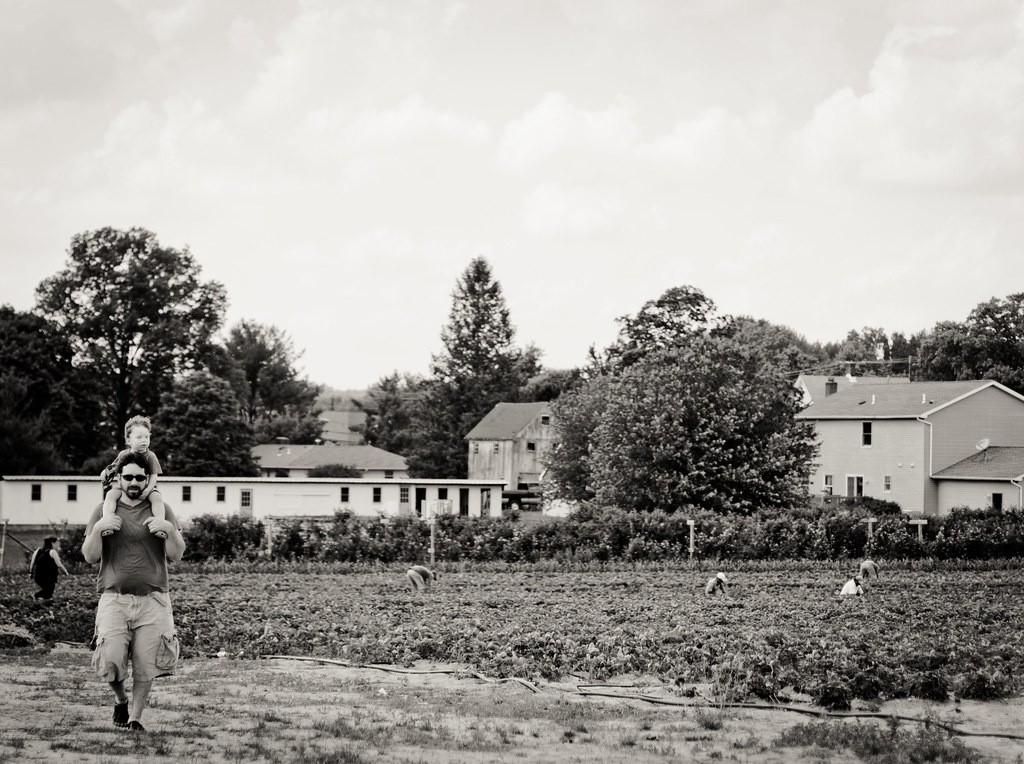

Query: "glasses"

xmin=121 ymin=474 xmax=148 ymax=482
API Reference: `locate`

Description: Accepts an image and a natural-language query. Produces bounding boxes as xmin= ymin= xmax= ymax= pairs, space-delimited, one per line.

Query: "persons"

xmin=99 ymin=416 xmax=166 ymax=538
xmin=407 ymin=566 xmax=436 ymax=591
xmin=81 ymin=451 xmax=185 ymax=731
xmin=705 ymin=572 xmax=727 ymax=596
xmin=28 ymin=536 xmax=68 ymax=599
xmin=841 ymin=576 xmax=864 ymax=595
xmin=861 ymin=561 xmax=880 ymax=579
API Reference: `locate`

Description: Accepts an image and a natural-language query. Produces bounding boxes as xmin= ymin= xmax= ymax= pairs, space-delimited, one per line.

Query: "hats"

xmin=717 ymin=572 xmax=728 ymax=582
xmin=856 ymin=576 xmax=864 ymax=584
xmin=433 ymin=571 xmax=439 ymax=581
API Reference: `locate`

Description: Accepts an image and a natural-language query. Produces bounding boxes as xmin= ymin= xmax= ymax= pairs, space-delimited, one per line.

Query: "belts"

xmin=121 ymin=587 xmax=161 ymax=595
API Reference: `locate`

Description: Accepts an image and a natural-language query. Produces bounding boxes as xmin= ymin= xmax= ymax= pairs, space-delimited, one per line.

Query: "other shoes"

xmin=156 ymin=531 xmax=167 ymax=539
xmin=112 ymin=701 xmax=130 ymax=727
xmin=128 ymin=721 xmax=144 ymax=732
xmin=102 ymin=529 xmax=113 ymax=536
xmin=31 ymin=594 xmax=37 ymax=603
